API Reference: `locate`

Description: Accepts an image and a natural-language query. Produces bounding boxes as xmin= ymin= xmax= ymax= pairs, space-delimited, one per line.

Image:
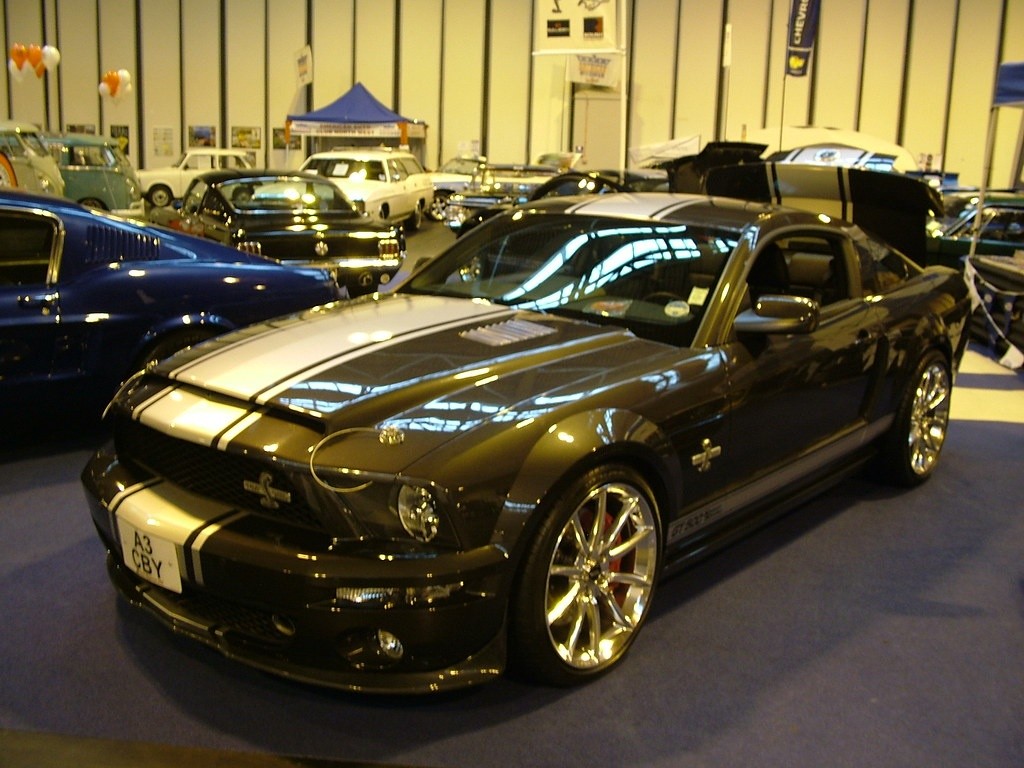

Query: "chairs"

xmin=597 ymin=240 xmax=835 ymax=309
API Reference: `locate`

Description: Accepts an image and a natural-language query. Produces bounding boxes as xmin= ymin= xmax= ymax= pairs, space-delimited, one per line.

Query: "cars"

xmin=81 ymin=131 xmax=973 ymax=699
xmin=430 ymin=155 xmax=487 ymax=222
xmin=0 ymin=118 xmax=65 ymax=197
xmin=926 ymin=192 xmax=1024 ymax=276
xmin=149 ymin=167 xmax=407 ymax=297
xmin=137 ymin=148 xmax=254 ymax=207
xmin=445 ymin=164 xmax=561 ymax=231
xmin=250 ymin=147 xmax=434 ymax=231
xmin=0 ymin=187 xmax=340 ymax=431
xmin=44 ymin=133 xmax=142 ymax=213
xmin=445 ymin=168 xmax=670 ymax=283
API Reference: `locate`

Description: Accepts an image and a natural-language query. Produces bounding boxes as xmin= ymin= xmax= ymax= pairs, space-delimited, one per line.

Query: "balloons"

xmin=8 ymin=42 xmax=62 ymax=83
xmin=99 ymin=69 xmax=132 ymax=104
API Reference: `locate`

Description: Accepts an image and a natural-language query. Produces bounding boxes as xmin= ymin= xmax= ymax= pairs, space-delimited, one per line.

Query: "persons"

xmin=231 ymin=186 xmax=253 ymax=204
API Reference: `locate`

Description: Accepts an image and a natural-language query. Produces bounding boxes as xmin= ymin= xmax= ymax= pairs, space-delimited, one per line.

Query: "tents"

xmin=285 ymin=82 xmax=428 ymax=171
xmin=964 ymin=62 xmax=1024 ymax=260
